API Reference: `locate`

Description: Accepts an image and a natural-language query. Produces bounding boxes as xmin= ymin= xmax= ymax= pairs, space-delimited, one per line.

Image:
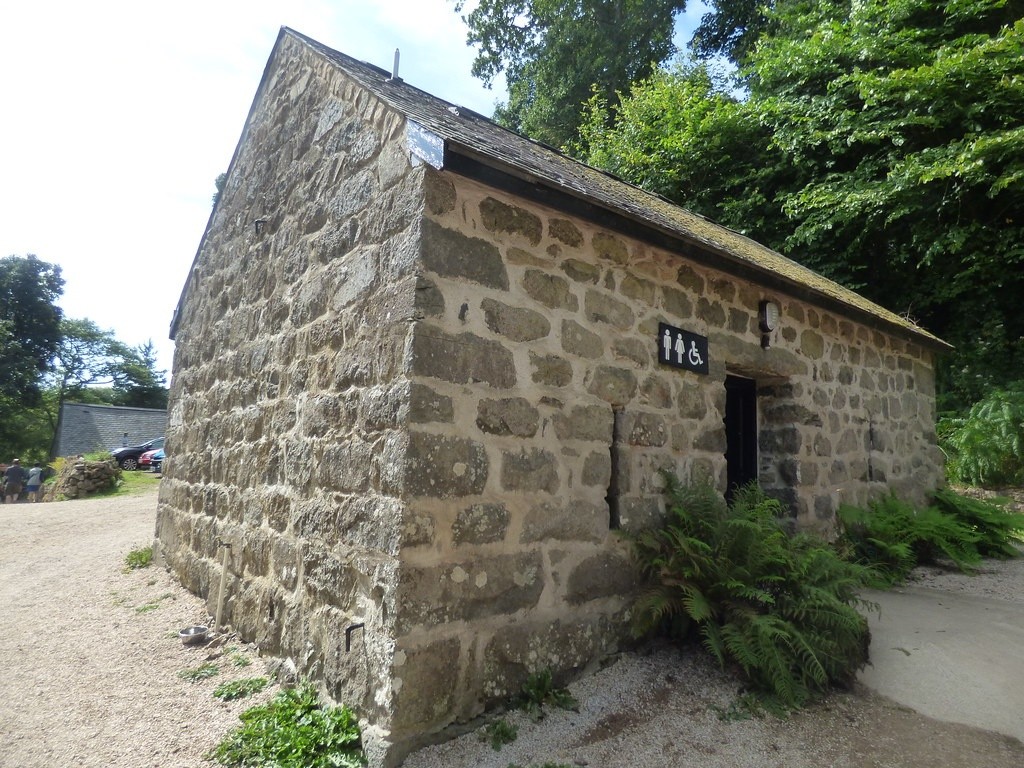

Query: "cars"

xmin=111 ymin=435 xmax=165 ymax=471
xmin=138 ymin=447 xmax=163 ymax=470
xmin=151 ymin=448 xmax=165 ymax=472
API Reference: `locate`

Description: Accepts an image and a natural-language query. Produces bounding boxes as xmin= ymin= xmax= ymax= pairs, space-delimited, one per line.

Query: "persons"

xmin=27 ymin=462 xmax=44 ymax=503
xmin=3 ymin=459 xmax=27 ymax=504
xmin=122 ymin=433 xmax=129 ymax=447
